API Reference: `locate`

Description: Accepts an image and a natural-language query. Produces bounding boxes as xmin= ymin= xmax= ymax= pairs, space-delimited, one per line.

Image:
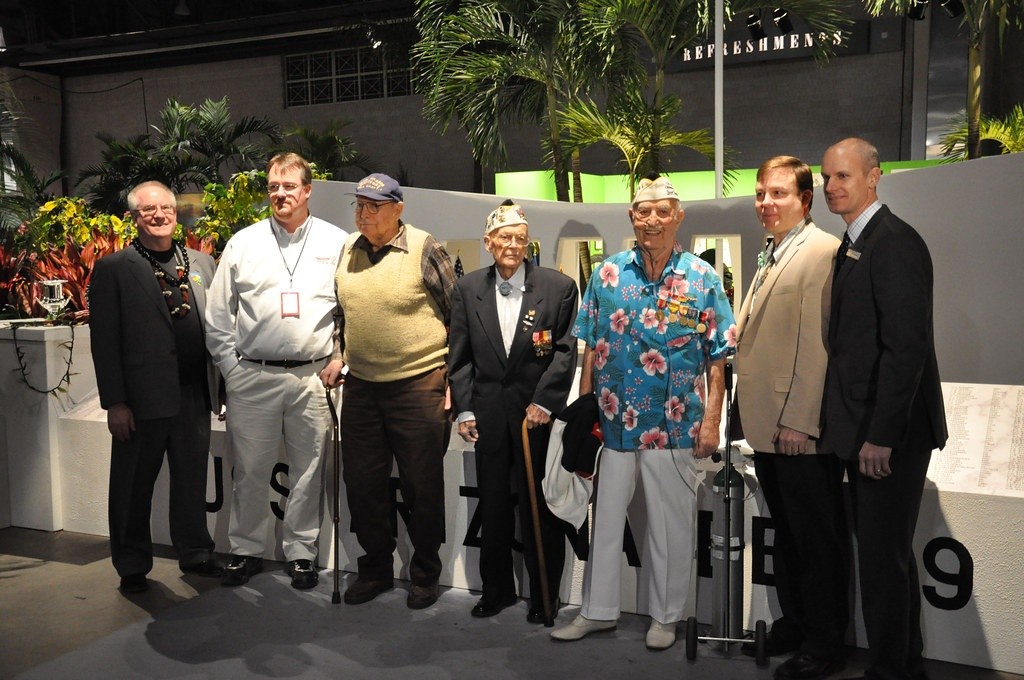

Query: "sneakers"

xmin=287 ymin=559 xmax=320 ymax=590
xmin=218 ymin=549 xmax=264 ymax=587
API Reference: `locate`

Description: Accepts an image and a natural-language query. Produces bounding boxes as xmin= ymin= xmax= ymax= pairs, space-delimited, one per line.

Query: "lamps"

xmin=907 ymin=0 xmax=965 ymax=22
xmin=362 ymin=26 xmax=382 ymax=49
xmin=746 ymin=8 xmax=793 ymax=43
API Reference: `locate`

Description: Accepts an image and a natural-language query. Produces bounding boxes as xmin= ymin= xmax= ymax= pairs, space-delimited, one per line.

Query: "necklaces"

xmin=150 ymin=246 xmax=191 ymax=318
xmin=133 ymin=236 xmax=190 ymax=287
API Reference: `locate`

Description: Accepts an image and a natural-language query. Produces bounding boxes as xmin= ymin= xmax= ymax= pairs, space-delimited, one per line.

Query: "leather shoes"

xmin=471 ymin=591 xmax=517 ymax=618
xmin=527 ymin=594 xmax=560 ymax=623
xmin=549 ymin=613 xmax=617 ymax=641
xmin=645 ymin=617 xmax=677 ymax=650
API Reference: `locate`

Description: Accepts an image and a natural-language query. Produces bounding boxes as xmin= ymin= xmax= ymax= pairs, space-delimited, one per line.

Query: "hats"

xmin=486 ymin=199 xmax=529 ymax=237
xmin=633 ymin=168 xmax=680 ymax=202
xmin=343 ymin=173 xmax=403 ymax=202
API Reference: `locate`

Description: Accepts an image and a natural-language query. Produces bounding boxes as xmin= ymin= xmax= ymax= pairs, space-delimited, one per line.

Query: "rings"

xmin=793 ymin=451 xmax=799 ymax=454
xmin=874 ymin=469 xmax=882 ymax=473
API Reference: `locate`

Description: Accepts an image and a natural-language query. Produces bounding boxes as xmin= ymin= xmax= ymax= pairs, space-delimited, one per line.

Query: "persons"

xmin=89 ymin=180 xmax=226 ymax=595
xmin=818 ymin=136 xmax=949 ymax=680
xmin=737 ymin=156 xmax=848 ymax=680
xmin=551 ymin=170 xmax=736 ymax=650
xmin=205 ymin=152 xmax=350 ymax=588
xmin=320 ymin=173 xmax=461 ymax=608
xmin=448 ymin=205 xmax=578 ymax=623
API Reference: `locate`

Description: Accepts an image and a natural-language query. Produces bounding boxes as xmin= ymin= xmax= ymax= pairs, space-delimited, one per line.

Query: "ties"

xmin=836 ymin=229 xmax=849 ymax=279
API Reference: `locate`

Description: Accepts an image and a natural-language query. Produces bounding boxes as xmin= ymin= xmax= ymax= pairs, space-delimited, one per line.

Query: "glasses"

xmin=265 ymin=183 xmax=306 ymax=193
xmin=491 ymin=230 xmax=530 ymax=247
xmin=351 ymin=201 xmax=394 ymax=214
xmin=132 ymin=203 xmax=177 ymax=215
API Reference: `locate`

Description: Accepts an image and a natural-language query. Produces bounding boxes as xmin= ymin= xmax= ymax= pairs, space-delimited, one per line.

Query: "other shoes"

xmin=192 ymin=553 xmax=225 ymax=578
xmin=742 ymin=630 xmax=802 ymax=657
xmin=120 ymin=571 xmax=152 ymax=601
xmin=344 ymin=574 xmax=394 ymax=605
xmin=407 ymin=579 xmax=439 ymax=609
xmin=773 ymin=647 xmax=846 ymax=680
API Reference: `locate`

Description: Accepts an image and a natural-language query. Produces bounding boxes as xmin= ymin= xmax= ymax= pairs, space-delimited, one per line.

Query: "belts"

xmin=243 ymin=351 xmax=332 ymax=368
